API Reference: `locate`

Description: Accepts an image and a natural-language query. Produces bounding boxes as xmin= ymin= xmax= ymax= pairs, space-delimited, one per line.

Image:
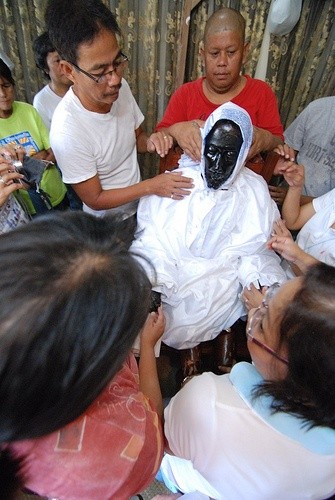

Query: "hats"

xmin=267 ymin=0 xmax=302 ymax=35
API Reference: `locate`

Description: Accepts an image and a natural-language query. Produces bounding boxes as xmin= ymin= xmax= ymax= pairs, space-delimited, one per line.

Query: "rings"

xmin=1 ymin=177 xmax=7 ymax=184
xmin=171 ymin=194 xmax=173 ymax=199
xmin=245 ymin=299 xmax=249 ymax=303
xmin=163 ymin=136 xmax=168 ymax=138
xmin=15 ymin=144 xmax=22 ymax=150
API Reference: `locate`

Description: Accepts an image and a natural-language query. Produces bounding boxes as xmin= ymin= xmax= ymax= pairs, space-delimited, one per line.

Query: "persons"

xmin=0 ymin=142 xmax=30 ymax=235
xmin=240 ymin=237 xmax=335 ymax=311
xmin=30 ymin=32 xmax=84 ymax=211
xmin=150 ymin=8 xmax=284 ymax=184
xmin=0 ymin=58 xmax=71 ymax=221
xmin=155 ymin=262 xmax=335 ymax=500
xmin=44 ymin=0 xmax=194 ymax=211
xmin=127 ymin=101 xmax=288 ymax=410
xmin=272 ymin=164 xmax=335 ymax=277
xmin=0 ymin=210 xmax=168 ymax=500
xmin=269 ymin=95 xmax=335 ymax=206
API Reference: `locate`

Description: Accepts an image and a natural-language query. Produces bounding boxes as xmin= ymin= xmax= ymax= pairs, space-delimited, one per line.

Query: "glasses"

xmin=64 ymin=52 xmax=130 ymax=86
xmin=245 ymin=281 xmax=294 ymax=369
